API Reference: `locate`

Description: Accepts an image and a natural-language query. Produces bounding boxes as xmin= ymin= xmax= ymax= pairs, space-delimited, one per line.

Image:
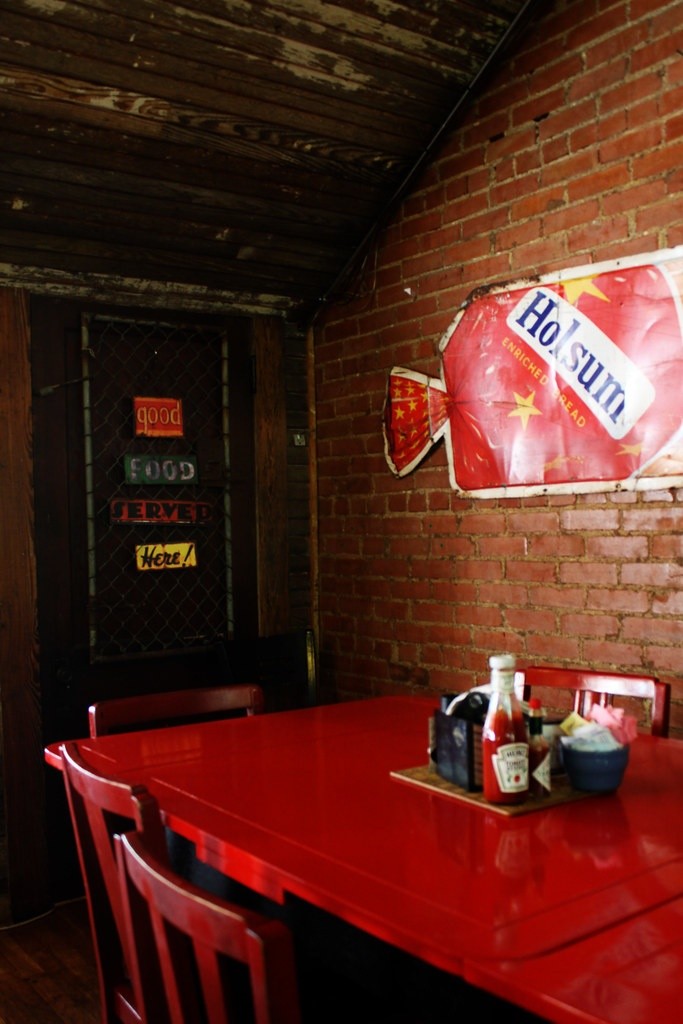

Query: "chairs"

xmin=111 ymin=829 xmax=301 ymax=1024
xmin=514 ymin=663 xmax=673 ymax=739
xmin=89 ymin=682 xmax=265 ymax=742
xmin=56 ymin=740 xmax=168 ymax=1024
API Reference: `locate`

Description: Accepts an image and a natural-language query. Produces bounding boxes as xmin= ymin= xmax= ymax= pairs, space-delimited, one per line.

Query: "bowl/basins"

xmin=559 ymin=740 xmax=628 ymax=795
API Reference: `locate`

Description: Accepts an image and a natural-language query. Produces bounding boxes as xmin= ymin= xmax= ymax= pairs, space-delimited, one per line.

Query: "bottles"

xmin=481 ymin=653 xmax=551 ymax=806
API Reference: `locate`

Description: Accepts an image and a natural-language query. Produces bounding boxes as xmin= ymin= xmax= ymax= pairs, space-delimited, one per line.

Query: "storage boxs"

xmin=432 ymin=705 xmax=544 ymax=794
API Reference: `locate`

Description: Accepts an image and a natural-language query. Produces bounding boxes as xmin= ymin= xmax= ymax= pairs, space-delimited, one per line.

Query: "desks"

xmin=42 ymin=694 xmax=683 ymax=1024
